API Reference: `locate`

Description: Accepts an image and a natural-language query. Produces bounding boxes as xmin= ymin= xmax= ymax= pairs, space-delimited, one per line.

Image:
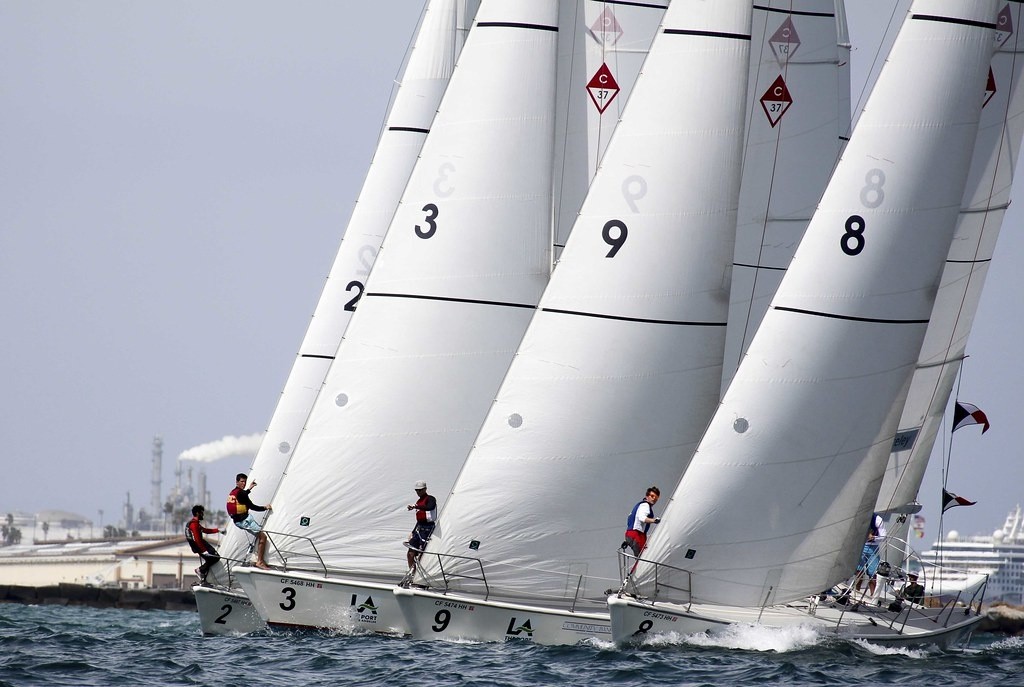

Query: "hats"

xmin=907 ymin=571 xmax=919 ymax=578
xmin=414 ymin=480 xmax=426 ymax=489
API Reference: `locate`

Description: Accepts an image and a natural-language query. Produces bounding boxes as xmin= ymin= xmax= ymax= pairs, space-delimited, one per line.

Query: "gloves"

xmin=654 ymin=518 xmax=661 ymax=523
xmin=218 ymin=528 xmax=226 ymax=533
xmin=202 ymin=551 xmax=208 ymax=555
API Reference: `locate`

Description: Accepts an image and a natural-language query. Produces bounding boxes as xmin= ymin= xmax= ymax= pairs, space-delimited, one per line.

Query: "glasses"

xmin=649 ymin=494 xmax=658 ymax=499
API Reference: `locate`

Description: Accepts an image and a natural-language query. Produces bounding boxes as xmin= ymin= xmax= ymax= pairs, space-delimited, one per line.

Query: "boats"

xmin=917 ymin=503 xmax=1024 ymax=604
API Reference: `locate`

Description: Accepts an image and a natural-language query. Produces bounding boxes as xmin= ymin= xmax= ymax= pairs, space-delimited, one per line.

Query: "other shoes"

xmin=195 ymin=568 xmax=204 ymax=580
xmin=201 ymin=582 xmax=213 ymax=588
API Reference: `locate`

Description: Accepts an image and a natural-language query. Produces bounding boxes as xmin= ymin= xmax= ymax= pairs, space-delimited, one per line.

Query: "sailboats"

xmin=192 ymin=0 xmax=1024 ymax=655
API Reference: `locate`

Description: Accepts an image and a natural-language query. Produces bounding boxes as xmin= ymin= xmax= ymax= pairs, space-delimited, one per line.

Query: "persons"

xmin=226 ymin=473 xmax=272 ymax=570
xmin=903 ymin=573 xmax=925 ymax=604
xmin=625 ymin=486 xmax=660 ymax=575
xmin=407 ymin=481 xmax=437 ymax=572
xmin=855 ymin=512 xmax=887 ymax=596
xmin=184 ymin=505 xmax=226 ymax=588
